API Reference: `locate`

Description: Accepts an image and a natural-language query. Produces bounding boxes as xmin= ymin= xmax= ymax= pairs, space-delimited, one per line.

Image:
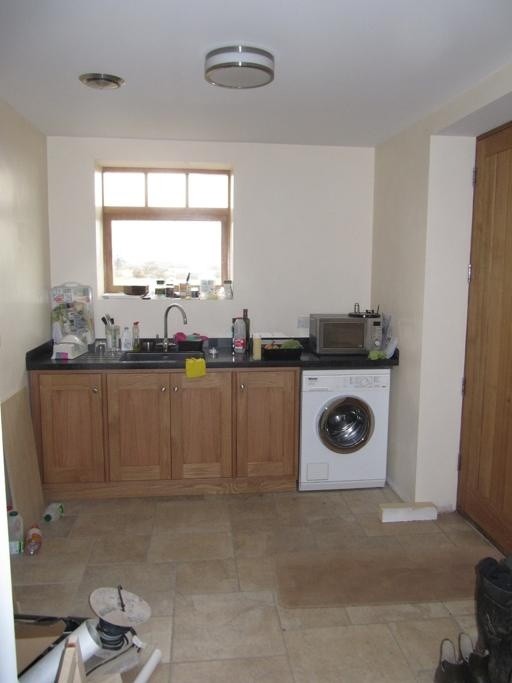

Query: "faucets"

xmin=156 ymin=303 xmax=188 ymax=352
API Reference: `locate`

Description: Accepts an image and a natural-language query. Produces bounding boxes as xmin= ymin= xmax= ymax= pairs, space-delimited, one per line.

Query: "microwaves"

xmin=308 ymin=312 xmax=383 ymax=355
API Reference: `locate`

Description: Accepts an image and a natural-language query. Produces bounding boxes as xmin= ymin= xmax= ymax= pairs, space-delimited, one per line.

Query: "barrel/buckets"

xmin=7 ymin=511 xmax=24 ymax=553
xmin=42 ymin=504 xmax=65 ymax=522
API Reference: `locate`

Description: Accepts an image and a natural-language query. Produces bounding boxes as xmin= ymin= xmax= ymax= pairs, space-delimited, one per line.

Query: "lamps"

xmin=202 ymin=39 xmax=276 ymax=89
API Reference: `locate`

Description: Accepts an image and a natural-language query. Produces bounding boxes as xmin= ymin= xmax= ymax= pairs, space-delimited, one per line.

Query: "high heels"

xmin=433 ymin=632 xmax=491 ymax=683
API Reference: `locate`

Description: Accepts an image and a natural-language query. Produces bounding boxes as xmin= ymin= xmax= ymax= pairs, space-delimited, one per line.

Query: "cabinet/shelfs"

xmin=30 ymin=368 xmax=107 ymax=501
xmin=235 ymin=365 xmax=301 ymax=495
xmin=108 ymin=368 xmax=233 ymax=499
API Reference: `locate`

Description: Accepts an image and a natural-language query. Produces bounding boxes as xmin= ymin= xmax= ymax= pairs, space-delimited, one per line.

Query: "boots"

xmin=473 ymin=556 xmax=512 ymax=683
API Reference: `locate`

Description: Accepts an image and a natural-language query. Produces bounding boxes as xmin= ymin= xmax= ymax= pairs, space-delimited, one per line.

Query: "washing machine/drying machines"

xmin=298 ymin=369 xmax=393 ymax=489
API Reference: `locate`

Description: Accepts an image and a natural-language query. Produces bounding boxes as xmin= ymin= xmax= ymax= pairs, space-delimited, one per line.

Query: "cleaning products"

xmin=132 ymin=320 xmax=142 ymax=352
xmin=121 ymin=327 xmax=132 ymax=350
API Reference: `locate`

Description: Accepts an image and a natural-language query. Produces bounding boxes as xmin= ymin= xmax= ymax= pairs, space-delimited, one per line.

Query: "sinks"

xmin=119 ymin=352 xmax=166 ymax=362
xmin=164 ymin=351 xmax=204 ymax=363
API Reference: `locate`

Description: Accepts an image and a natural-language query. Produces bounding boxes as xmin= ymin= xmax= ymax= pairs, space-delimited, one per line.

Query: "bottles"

xmin=120 ymin=320 xmax=141 ymax=352
xmin=154 ymin=279 xmax=233 ymax=299
xmin=42 ymin=501 xmax=63 ymax=522
xmin=6 ymin=505 xmax=23 ymax=556
xmin=23 ymin=523 xmax=42 ymax=555
xmin=233 ymin=308 xmax=250 ymax=353
